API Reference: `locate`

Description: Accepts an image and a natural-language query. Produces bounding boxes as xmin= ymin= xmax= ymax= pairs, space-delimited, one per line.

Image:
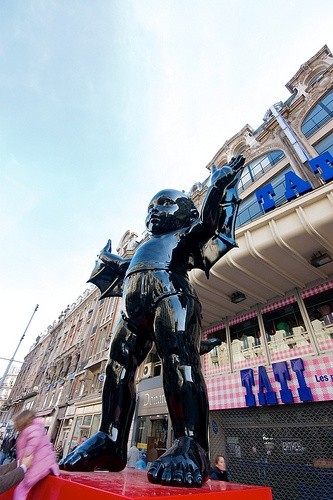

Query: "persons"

xmin=0 ymin=454 xmax=36 ymax=495
xmin=207 ymin=456 xmax=230 ymax=483
xmin=10 ymin=408 xmax=60 ymax=499
xmin=145 ymin=443 xmax=159 ymax=472
xmin=56 ymin=440 xmax=65 ymax=460
xmin=55 ymin=153 xmax=245 ymax=490
xmin=69 ymin=436 xmax=86 ymax=450
xmin=127 ymin=441 xmax=140 ymax=467
xmin=257 ymin=436 xmax=280 ymax=464
xmin=0 ymin=422 xmax=18 ymax=463
